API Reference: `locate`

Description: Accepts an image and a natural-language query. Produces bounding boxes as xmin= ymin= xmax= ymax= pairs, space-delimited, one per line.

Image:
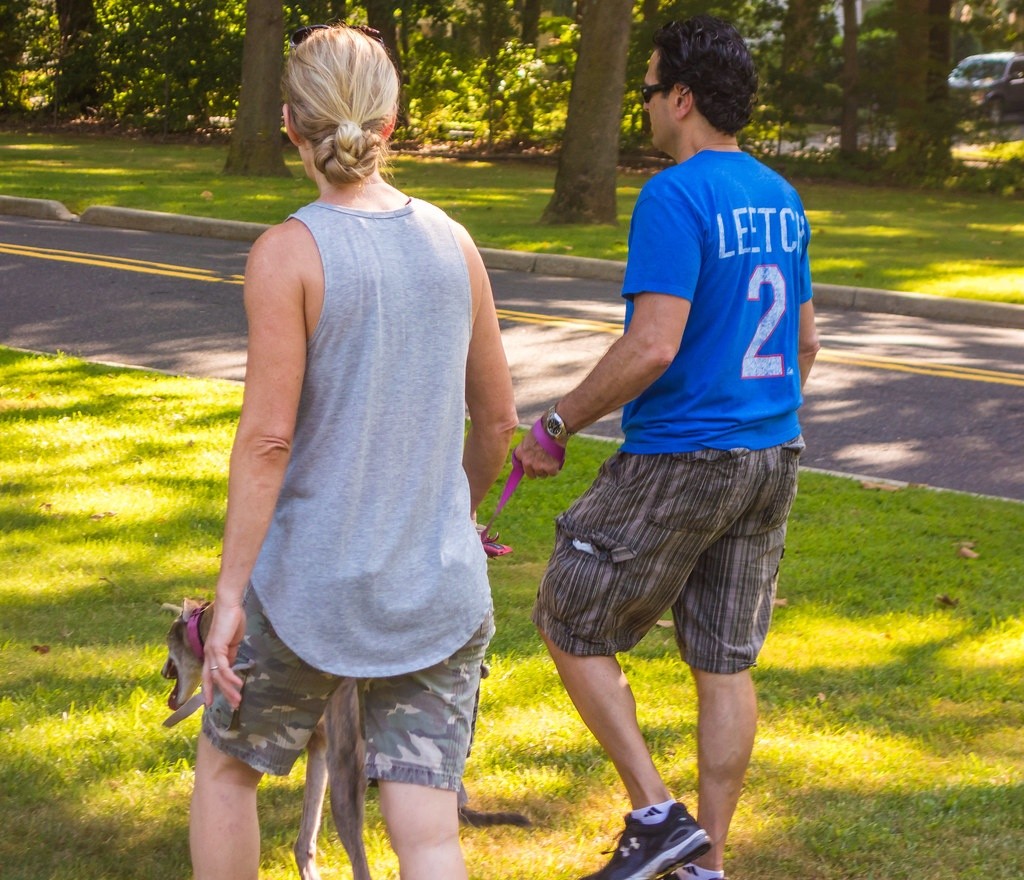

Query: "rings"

xmin=209 ymin=666 xmax=219 ymax=670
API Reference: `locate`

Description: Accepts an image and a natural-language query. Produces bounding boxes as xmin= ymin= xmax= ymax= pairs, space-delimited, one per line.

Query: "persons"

xmin=188 ymin=27 xmax=519 ymax=880
xmin=514 ymin=14 xmax=821 ymax=880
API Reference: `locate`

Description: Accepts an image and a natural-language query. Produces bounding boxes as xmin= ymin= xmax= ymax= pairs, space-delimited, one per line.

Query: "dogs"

xmin=161 ymin=596 xmax=534 ymax=880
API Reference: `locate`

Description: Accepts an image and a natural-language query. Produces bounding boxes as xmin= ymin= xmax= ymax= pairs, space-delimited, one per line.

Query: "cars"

xmin=947 ymin=49 xmax=1024 ymax=125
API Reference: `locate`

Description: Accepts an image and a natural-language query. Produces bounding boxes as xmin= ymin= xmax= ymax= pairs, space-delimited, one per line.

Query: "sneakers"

xmin=584 ymin=801 xmax=711 ymax=880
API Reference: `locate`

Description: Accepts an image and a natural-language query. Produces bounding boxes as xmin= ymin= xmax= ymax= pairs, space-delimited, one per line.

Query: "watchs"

xmin=546 ymin=404 xmax=574 ymax=440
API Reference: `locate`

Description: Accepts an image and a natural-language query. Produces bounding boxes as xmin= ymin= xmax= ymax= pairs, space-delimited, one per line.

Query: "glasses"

xmin=288 ymin=22 xmax=388 ymax=55
xmin=643 ymin=80 xmax=670 ymax=99
